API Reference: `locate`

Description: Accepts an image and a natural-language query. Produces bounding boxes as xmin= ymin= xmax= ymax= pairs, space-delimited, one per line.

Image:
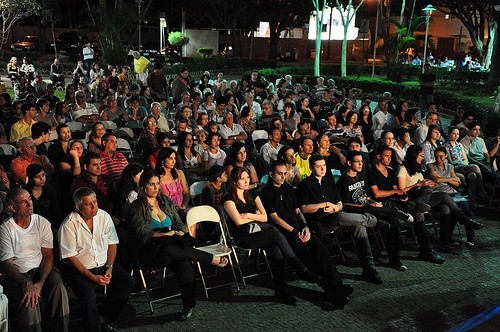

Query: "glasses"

xmin=384 ymin=137 xmax=394 ymax=141
xmin=353 ymin=160 xmax=364 ymax=163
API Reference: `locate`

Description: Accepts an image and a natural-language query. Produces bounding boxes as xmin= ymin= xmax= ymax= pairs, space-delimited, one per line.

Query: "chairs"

xmin=0 ymin=104 xmax=500 ymax=314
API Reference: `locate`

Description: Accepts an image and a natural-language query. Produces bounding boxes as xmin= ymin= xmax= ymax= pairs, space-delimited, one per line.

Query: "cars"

xmin=11 ymin=35 xmax=50 ymax=55
xmin=44 ymin=30 xmax=100 ymax=61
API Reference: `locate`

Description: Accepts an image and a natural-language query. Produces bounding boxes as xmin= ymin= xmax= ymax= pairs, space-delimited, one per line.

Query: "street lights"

xmin=134 ymin=0 xmax=145 ymax=50
xmin=421 ymin=4 xmax=437 ymax=74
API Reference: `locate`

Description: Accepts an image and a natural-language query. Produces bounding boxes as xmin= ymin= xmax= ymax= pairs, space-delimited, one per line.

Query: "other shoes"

xmin=425 ymin=252 xmax=446 ymax=264
xmin=466 ymin=241 xmax=475 ymax=246
xmin=362 ymin=268 xmax=379 ymax=276
xmin=413 ymin=202 xmax=431 ymax=212
xmin=300 ymin=269 xmax=322 ymax=283
xmin=376 ymin=219 xmax=391 ymax=228
xmin=181 ymin=307 xmax=193 ymax=320
xmin=102 ymin=319 xmax=117 ymax=331
xmin=465 ymin=219 xmax=484 ymax=230
xmin=273 ymin=292 xmax=298 ymax=305
xmin=216 ymin=257 xmax=228 ymax=268
xmin=446 ymin=241 xmax=460 ymax=247
xmin=324 ymin=283 xmax=354 ymax=300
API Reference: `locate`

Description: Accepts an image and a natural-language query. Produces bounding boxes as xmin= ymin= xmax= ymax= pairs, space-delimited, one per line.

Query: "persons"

xmin=57 ymin=186 xmax=130 ymax=332
xmin=0 ymin=189 xmax=64 ymax=332
xmin=0 ymin=57 xmax=500 ymax=320
xmin=411 ymin=52 xmax=481 ymax=71
xmin=83 ymin=42 xmax=94 ymax=78
xmin=132 ymin=51 xmax=150 ymax=88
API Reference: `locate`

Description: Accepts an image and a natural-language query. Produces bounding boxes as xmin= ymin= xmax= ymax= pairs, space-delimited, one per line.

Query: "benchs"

xmin=0 ymin=77 xmax=54 ymax=85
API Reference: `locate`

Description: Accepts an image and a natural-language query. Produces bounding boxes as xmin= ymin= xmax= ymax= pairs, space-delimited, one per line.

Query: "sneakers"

xmin=393 ymin=207 xmax=414 ymax=223
xmin=389 ymin=261 xmax=407 ymax=271
